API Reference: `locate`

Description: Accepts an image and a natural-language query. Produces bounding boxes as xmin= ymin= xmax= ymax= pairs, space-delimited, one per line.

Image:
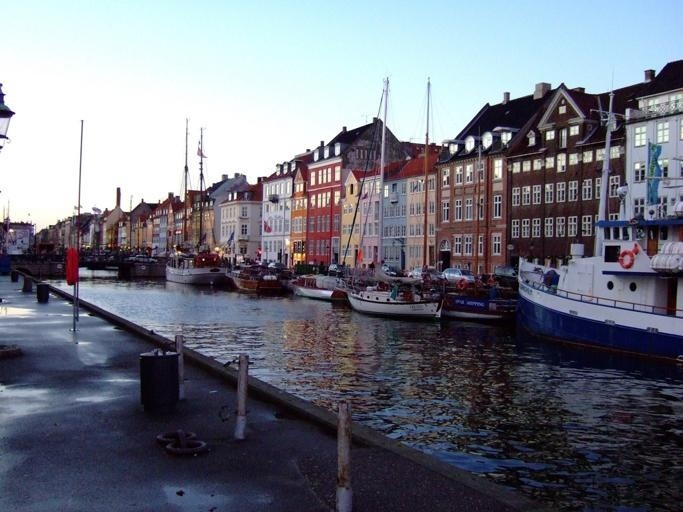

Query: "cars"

xmin=442 ymin=266 xmax=479 ymax=284
xmin=81 ymin=254 xmax=158 ymax=264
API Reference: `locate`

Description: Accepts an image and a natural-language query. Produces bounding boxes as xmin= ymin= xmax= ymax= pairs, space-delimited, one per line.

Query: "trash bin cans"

xmin=11 ymin=270 xmax=19 ymax=282
xmin=37 ymin=283 xmax=49 ymax=304
xmin=21 ymin=277 xmax=33 ymax=291
xmin=139 ymin=349 xmax=180 ymax=415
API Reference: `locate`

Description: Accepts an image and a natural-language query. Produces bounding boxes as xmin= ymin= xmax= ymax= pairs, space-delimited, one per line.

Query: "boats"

xmin=443 ymin=290 xmax=518 ymax=322
xmin=513 ymin=61 xmax=683 ymax=366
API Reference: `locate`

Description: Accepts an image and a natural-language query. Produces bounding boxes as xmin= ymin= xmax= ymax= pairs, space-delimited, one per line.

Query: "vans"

xmin=493 ymin=264 xmax=519 ymax=289
